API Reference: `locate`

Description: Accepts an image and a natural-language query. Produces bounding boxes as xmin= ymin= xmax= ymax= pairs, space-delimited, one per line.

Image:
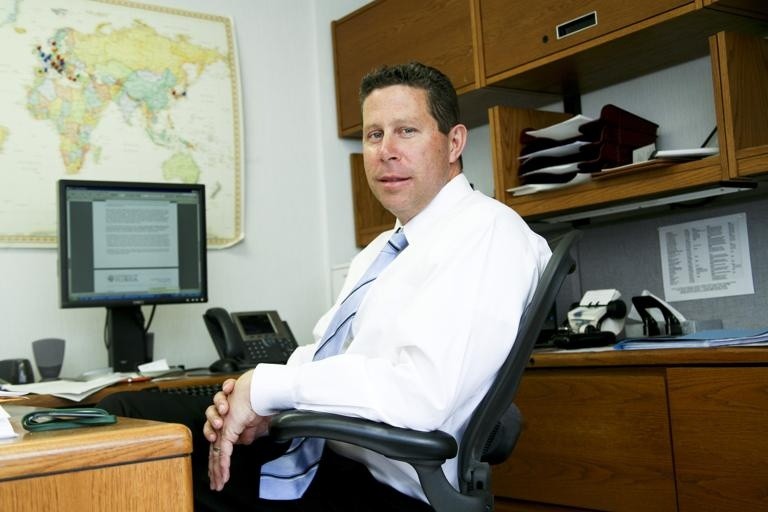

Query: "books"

xmin=610 ymin=326 xmax=768 ymax=350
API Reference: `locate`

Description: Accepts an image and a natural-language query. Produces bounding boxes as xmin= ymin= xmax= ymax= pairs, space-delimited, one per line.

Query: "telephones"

xmin=202 ymin=308 xmax=299 ymax=372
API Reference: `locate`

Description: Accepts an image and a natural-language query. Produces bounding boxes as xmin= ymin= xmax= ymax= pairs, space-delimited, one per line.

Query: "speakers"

xmin=32 ymin=338 xmax=65 ymax=381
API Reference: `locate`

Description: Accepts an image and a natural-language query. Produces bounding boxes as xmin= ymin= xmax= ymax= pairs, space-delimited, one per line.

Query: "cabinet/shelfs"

xmin=331 ymin=0 xmax=766 ymax=512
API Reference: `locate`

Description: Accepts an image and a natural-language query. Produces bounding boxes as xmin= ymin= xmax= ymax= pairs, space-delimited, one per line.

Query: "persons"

xmin=91 ymin=60 xmax=554 ymax=510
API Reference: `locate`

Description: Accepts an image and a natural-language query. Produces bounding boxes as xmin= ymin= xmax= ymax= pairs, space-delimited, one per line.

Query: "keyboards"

xmin=140 ymin=383 xmax=223 ymax=395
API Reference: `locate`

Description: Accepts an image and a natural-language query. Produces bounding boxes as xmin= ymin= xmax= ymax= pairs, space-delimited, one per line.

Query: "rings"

xmin=211 ymin=444 xmax=221 ymax=453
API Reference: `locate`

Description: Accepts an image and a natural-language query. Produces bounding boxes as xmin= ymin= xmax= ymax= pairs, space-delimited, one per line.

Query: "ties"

xmin=256 ymin=225 xmax=409 ymax=501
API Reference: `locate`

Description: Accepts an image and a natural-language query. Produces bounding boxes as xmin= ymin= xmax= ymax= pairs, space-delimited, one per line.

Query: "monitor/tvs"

xmin=57 ymin=178 xmax=208 ymax=373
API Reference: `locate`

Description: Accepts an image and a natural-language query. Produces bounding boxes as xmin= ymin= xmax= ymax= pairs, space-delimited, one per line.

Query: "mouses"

xmin=209 ymin=358 xmax=241 ymax=374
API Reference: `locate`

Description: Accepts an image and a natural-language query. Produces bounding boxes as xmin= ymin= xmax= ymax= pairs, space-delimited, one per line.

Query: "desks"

xmin=0 ymin=367 xmax=248 ymax=511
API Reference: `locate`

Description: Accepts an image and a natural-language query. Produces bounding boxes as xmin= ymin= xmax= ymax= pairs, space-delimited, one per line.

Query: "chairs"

xmin=265 ymin=229 xmax=584 ymax=510
